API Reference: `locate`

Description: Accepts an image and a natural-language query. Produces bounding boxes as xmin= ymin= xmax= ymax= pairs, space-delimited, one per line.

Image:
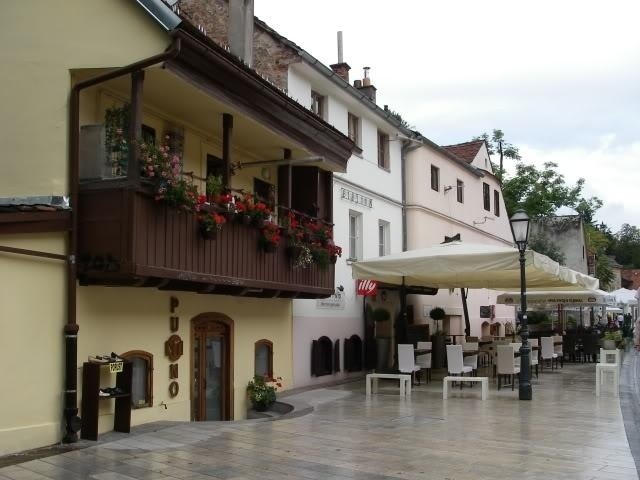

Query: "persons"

xmin=551 ymin=312 xmax=633 ymax=363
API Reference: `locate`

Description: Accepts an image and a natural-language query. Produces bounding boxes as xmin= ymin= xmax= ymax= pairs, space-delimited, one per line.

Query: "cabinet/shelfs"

xmin=81 ymin=361 xmax=133 ymax=441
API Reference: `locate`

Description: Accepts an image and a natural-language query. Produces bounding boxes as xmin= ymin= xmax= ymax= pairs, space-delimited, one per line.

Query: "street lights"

xmin=511 ymin=207 xmax=536 ymax=402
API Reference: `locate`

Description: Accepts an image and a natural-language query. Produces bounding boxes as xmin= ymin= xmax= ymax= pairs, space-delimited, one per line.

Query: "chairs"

xmin=397 ymin=335 xmax=563 ymax=392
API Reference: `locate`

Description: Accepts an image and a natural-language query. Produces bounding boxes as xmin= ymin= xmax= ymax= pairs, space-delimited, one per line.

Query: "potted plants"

xmin=602 ymin=330 xmax=623 ymax=350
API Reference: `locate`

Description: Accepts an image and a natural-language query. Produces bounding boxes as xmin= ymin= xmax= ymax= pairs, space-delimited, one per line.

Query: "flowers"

xmin=264 ymin=375 xmax=282 ymax=401
xmin=249 ymin=203 xmax=271 ymax=219
xmin=288 ymin=221 xmax=305 ymax=248
xmin=199 ymin=211 xmax=225 ymax=231
xmin=259 ymin=222 xmax=281 ymax=246
xmin=247 ymin=374 xmax=267 ymax=410
xmin=326 ymin=243 xmax=342 ymax=256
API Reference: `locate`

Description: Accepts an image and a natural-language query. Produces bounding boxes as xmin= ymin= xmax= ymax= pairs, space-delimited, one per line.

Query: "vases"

xmin=258 ymin=219 xmax=265 ymax=225
xmin=269 ymin=394 xmax=276 ymax=405
xmin=254 ymin=401 xmax=264 ymax=412
xmin=203 ymin=230 xmax=220 ymax=241
xmin=286 ymin=247 xmax=303 ymax=257
xmin=265 ymin=246 xmax=275 ymax=253
xmin=330 ymin=256 xmax=337 ymax=264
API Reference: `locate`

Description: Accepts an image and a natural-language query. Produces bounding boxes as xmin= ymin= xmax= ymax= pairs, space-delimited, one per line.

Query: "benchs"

xmin=365 ymin=373 xmax=412 ymax=395
xmin=442 ymin=376 xmax=489 ymax=400
